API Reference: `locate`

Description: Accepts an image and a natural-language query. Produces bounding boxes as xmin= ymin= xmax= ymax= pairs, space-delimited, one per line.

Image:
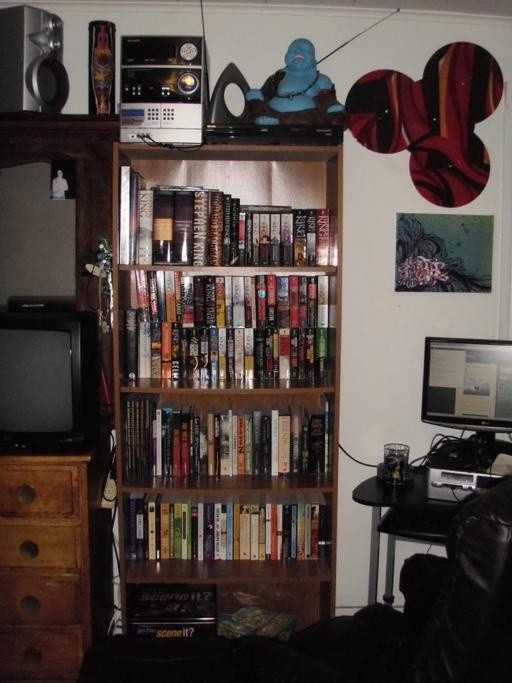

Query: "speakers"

xmin=0 ymin=5 xmax=69 ymax=114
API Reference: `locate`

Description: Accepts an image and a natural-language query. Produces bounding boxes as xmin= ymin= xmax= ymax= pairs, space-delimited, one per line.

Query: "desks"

xmin=352 ymin=469 xmax=495 ymax=606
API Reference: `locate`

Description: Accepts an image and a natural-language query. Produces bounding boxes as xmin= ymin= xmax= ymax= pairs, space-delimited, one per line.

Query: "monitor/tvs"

xmin=420 ymin=336 xmax=512 ymax=442
xmin=0 ymin=305 xmax=99 ymax=456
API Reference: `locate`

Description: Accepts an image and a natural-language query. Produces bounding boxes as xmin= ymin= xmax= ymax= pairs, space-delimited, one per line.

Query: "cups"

xmin=383 ymin=443 xmax=410 ymax=485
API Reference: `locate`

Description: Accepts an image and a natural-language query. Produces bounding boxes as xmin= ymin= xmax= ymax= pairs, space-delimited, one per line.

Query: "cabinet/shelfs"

xmin=0 ymin=111 xmax=118 ymax=457
xmin=113 ymin=143 xmax=344 ymax=635
xmin=0 ymin=458 xmax=97 ymax=682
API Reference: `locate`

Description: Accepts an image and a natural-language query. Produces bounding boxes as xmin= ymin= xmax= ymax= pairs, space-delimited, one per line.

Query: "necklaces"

xmin=274 ymin=70 xmax=320 ymax=98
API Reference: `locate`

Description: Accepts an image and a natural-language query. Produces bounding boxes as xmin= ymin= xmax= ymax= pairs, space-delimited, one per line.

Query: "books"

xmin=123 ymin=391 xmax=333 ymax=474
xmin=122 ymin=491 xmax=322 ymax=560
xmin=119 ymin=165 xmax=335 ymax=266
xmin=126 ymin=271 xmax=327 ymax=379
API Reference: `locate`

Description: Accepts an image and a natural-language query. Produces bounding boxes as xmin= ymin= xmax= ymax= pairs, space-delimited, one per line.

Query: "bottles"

xmin=91 ymin=25 xmax=114 ymax=114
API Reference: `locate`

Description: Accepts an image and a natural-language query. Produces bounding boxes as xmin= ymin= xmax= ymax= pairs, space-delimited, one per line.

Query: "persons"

xmin=247 ymin=38 xmax=346 ymax=129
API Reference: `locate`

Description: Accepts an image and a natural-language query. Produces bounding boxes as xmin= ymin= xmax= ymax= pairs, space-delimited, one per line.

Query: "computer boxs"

xmin=426 ymin=459 xmax=505 ymax=504
